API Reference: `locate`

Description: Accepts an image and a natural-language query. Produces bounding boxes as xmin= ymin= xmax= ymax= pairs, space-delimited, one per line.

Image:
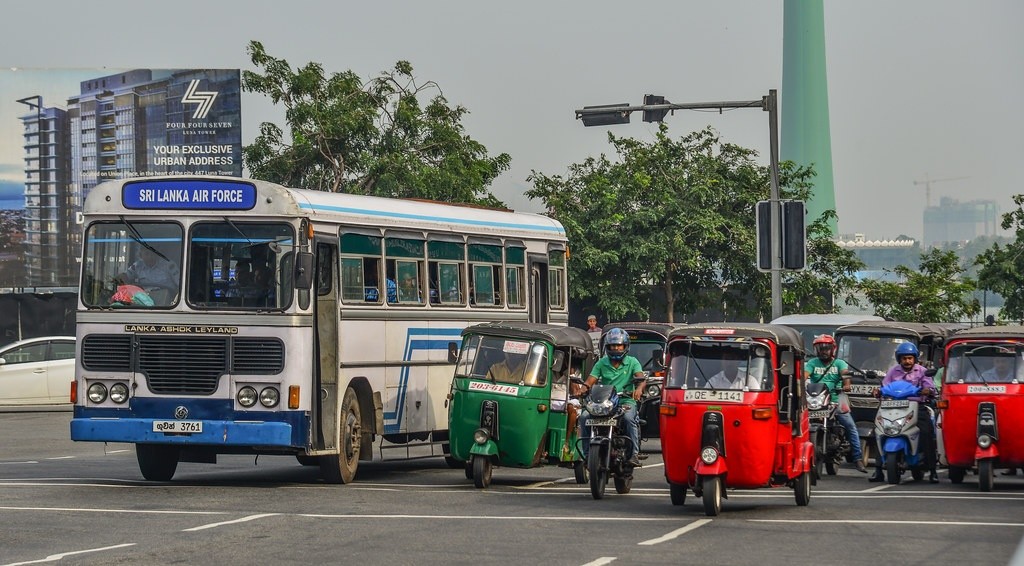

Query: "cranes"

xmin=913 ymin=174 xmax=973 ymax=206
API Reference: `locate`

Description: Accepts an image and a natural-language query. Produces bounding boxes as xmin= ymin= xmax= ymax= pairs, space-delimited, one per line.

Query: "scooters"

xmin=863 ymin=362 xmax=940 ymax=486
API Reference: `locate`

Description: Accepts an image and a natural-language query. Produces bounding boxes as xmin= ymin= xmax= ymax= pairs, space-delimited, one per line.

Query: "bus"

xmin=66 ymin=170 xmax=574 ymax=483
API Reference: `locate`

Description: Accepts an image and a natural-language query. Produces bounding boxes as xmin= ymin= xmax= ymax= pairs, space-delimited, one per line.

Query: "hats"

xmin=588 ymin=315 xmax=596 ymax=319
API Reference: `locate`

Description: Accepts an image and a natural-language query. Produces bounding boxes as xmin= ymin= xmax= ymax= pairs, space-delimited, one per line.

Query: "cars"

xmin=0 ymin=335 xmax=80 ymax=413
xmin=762 ymin=312 xmax=890 ymax=384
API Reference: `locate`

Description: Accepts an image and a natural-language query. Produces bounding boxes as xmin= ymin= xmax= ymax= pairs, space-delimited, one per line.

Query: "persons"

xmin=978 ymin=356 xmax=1023 ymax=381
xmin=704 ymin=346 xmax=760 ymax=389
xmin=586 ymin=315 xmax=602 ymax=332
xmin=573 ymin=328 xmax=646 ymax=465
xmin=804 ymin=333 xmax=869 ymax=473
xmin=486 ymin=346 xmax=582 ymax=466
xmin=225 ymin=263 xmax=422 ymax=308
xmin=111 ymin=242 xmax=181 ymax=292
xmin=860 ymin=341 xmax=940 ymax=483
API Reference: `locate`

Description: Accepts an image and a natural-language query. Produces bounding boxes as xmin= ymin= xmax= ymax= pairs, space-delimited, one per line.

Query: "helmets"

xmin=605 ymin=328 xmax=631 ymax=361
xmin=896 ymin=342 xmax=920 ymax=358
xmin=812 ymin=334 xmax=836 ymax=349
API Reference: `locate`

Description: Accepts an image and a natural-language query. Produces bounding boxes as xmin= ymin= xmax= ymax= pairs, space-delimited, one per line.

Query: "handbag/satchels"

xmin=838 ymin=389 xmax=850 ymax=414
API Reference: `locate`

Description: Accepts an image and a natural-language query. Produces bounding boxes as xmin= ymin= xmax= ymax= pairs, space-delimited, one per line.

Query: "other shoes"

xmin=628 ymin=456 xmax=640 ymax=463
xmin=584 ymin=457 xmax=588 ymax=464
xmin=564 ymin=448 xmax=569 ymax=452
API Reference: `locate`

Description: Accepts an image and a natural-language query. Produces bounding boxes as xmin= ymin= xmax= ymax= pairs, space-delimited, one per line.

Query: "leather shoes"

xmin=868 ymin=471 xmax=884 ymax=481
xmin=930 ymin=473 xmax=938 ymax=482
xmin=856 ymin=462 xmax=868 ymax=472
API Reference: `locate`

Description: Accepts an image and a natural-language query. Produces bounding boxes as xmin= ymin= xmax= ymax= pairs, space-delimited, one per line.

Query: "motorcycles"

xmin=797 ymin=380 xmax=847 ymax=483
xmin=568 ymin=372 xmax=648 ymax=500
xmin=653 ymin=323 xmax=817 ymax=518
xmin=586 ymin=321 xmax=687 ymax=438
xmin=933 ymin=321 xmax=1024 ymax=492
xmin=445 ymin=321 xmax=597 ymax=489
xmin=827 ymin=319 xmax=971 ymax=470
